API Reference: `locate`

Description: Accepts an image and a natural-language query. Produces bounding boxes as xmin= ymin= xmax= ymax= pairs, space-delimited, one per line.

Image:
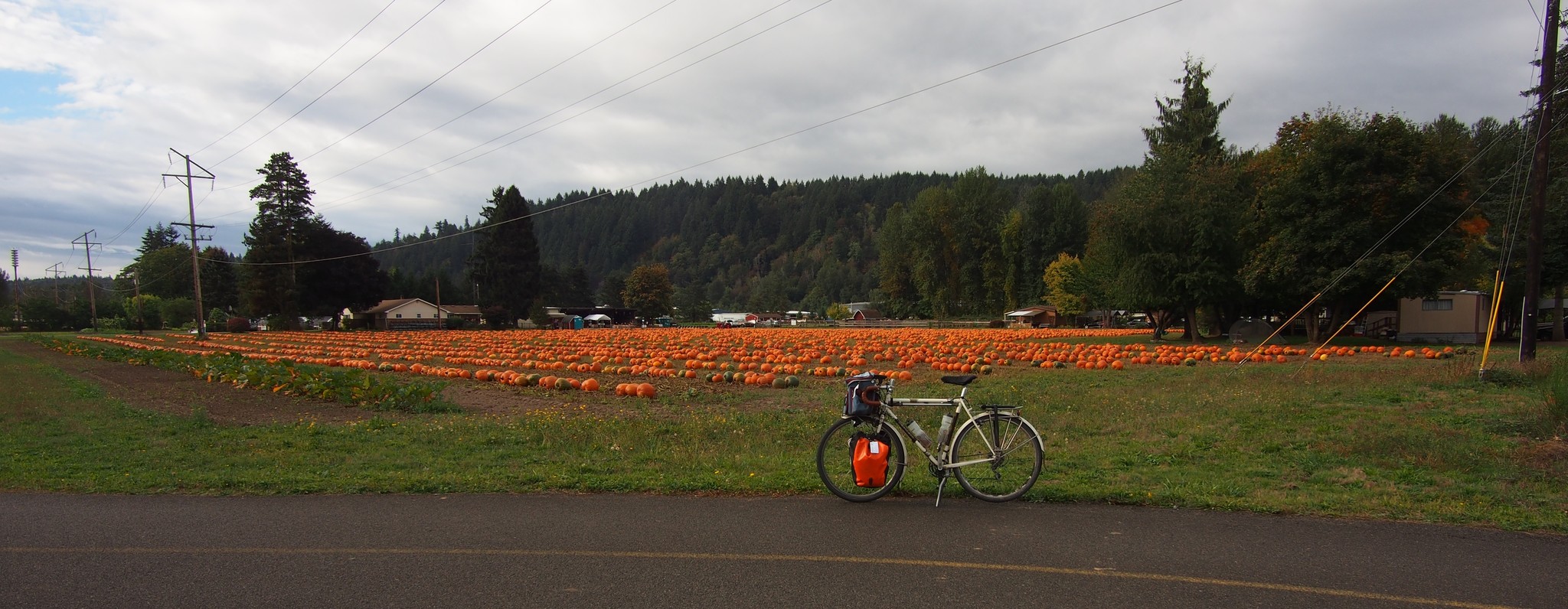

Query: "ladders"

xmin=1359 ymin=318 xmax=1368 ymax=336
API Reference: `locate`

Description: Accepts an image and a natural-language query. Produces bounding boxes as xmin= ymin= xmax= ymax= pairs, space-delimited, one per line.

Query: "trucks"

xmin=724 ymin=319 xmax=751 ymax=328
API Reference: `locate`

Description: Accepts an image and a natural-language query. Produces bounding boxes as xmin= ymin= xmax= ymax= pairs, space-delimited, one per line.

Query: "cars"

xmin=745 ymin=318 xmax=782 ymax=328
xmin=1541 ymin=320 xmax=1568 ymax=342
xmin=1126 ymin=318 xmax=1152 ymax=329
xmin=1083 ymin=319 xmax=1104 ymax=329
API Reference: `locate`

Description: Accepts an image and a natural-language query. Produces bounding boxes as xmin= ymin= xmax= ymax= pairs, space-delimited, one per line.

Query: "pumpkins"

xmin=77 ymin=327 xmax=1476 ymax=398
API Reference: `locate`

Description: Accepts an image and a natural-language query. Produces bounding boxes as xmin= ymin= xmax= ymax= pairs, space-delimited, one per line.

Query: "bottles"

xmin=906 ymin=418 xmax=933 ymax=448
xmin=936 ymin=412 xmax=953 ymax=446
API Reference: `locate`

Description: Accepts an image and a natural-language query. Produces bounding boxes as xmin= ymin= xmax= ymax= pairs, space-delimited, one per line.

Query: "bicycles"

xmin=815 ymin=373 xmax=1049 ymax=507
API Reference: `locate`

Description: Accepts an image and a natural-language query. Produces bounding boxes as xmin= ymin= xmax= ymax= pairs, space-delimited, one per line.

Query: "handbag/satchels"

xmin=847 ymin=431 xmax=890 ymax=490
